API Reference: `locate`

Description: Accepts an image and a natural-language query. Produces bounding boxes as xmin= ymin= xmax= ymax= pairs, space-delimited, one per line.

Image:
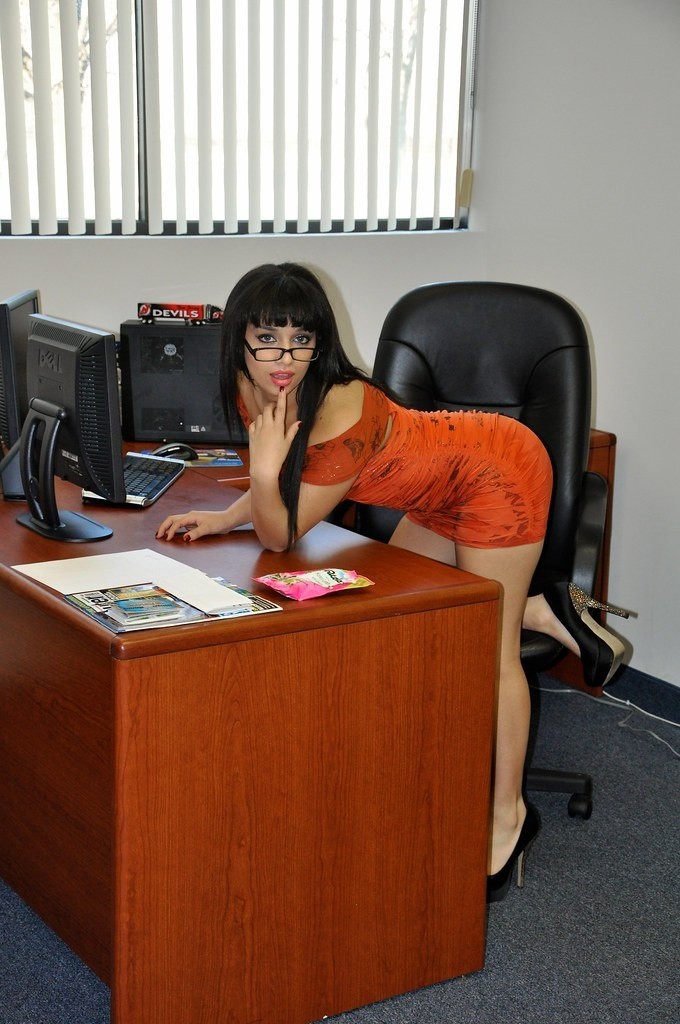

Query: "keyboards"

xmin=81 ymin=451 xmax=185 ymax=508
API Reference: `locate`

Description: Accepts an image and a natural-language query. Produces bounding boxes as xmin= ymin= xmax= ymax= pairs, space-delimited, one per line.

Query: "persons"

xmin=154 ymin=261 xmax=630 ymax=904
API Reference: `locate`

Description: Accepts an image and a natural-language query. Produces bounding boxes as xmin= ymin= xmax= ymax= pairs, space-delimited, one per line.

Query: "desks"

xmin=0 ymin=430 xmax=618 ymax=1024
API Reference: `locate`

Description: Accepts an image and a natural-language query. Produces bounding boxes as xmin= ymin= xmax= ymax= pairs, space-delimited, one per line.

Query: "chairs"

xmin=351 ymin=281 xmax=609 ymax=821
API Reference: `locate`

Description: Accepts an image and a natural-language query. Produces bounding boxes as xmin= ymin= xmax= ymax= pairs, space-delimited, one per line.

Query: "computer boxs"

xmin=119 ymin=319 xmax=250 ymax=446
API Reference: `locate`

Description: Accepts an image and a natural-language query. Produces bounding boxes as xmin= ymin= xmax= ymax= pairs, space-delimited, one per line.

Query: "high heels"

xmin=543 ymin=581 xmax=629 ymax=687
xmin=485 ymin=801 xmax=542 ymax=903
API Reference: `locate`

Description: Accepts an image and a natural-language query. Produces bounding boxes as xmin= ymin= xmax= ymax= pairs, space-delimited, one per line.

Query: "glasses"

xmin=243 ymin=337 xmax=320 ymax=362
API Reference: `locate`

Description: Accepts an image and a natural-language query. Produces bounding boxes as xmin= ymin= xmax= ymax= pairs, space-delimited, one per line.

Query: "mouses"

xmin=149 ymin=443 xmax=198 ymax=461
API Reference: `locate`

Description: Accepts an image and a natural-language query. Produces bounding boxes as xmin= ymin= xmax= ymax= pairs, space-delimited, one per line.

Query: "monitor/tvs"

xmin=0 ymin=289 xmax=127 ymax=542
